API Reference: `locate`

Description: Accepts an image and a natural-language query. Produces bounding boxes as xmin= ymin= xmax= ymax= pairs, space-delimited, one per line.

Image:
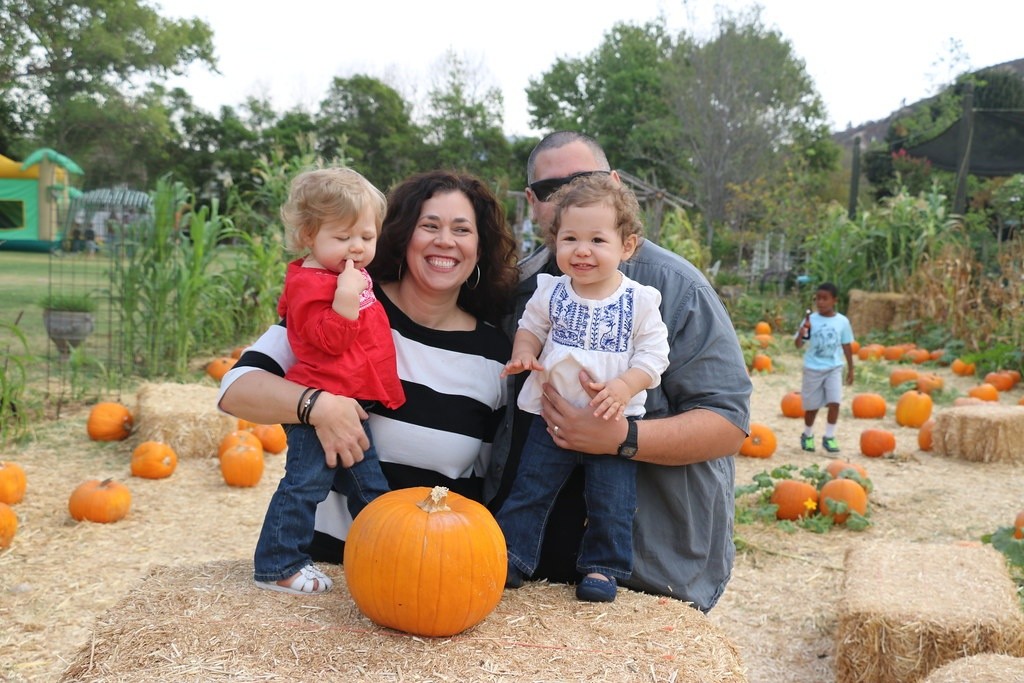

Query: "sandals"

xmin=255 ymin=563 xmax=332 ymax=595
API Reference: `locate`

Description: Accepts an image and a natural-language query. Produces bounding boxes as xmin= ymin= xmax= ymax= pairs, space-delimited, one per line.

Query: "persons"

xmin=494 ymin=172 xmax=670 ymax=602
xmin=793 ymin=281 xmax=854 ymax=452
xmin=70 ymin=209 xmax=138 ymax=254
xmin=501 ymin=128 xmax=752 ymax=617
xmin=253 ymin=170 xmax=405 ymax=595
xmin=216 ymin=170 xmax=514 ymax=567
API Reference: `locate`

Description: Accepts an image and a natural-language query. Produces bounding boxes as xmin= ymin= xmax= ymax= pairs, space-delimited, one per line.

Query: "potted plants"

xmin=35 ymin=291 xmax=98 ymax=362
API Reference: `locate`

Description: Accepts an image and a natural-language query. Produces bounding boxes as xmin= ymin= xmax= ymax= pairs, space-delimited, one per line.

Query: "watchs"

xmin=618 ymin=417 xmax=638 ymax=460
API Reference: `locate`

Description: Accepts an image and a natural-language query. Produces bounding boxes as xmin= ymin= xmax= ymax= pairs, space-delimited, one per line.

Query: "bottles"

xmin=803 ymin=311 xmax=812 ymax=340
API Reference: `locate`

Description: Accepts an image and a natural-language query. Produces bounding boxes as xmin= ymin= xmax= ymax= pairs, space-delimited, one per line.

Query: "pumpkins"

xmin=218 ymin=418 xmax=287 ymax=487
xmin=131 ymin=441 xmax=177 ymax=479
xmin=207 ymin=344 xmax=249 ymax=379
xmin=68 ymin=476 xmax=130 ymax=523
xmin=734 ymin=321 xmax=1024 ymax=524
xmin=343 ymin=486 xmax=508 ymax=638
xmin=0 ymin=460 xmax=27 ymax=553
xmin=1013 ymin=510 xmax=1024 ymax=539
xmin=87 ymin=403 xmax=132 ymax=441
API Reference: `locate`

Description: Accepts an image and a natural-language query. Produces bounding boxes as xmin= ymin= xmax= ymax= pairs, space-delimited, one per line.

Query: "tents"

xmin=83 ymin=187 xmax=154 ymax=210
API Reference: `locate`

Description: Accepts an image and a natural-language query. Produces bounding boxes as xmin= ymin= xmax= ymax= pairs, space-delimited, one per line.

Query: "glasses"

xmin=530 ymin=170 xmax=611 ymax=202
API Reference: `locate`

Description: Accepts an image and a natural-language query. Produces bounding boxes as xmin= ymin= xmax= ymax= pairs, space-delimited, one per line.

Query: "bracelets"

xmin=297 ymin=386 xmax=325 ymax=427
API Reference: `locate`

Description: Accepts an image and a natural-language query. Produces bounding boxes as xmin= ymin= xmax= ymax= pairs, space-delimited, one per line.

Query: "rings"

xmin=553 ymin=427 xmax=559 ymax=436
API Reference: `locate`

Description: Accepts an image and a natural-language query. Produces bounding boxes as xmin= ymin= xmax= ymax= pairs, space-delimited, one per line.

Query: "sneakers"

xmin=823 ymin=437 xmax=840 ymax=451
xmin=801 ymin=432 xmax=815 ymax=451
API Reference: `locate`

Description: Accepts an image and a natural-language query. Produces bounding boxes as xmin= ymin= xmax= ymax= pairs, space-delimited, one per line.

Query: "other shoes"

xmin=575 ymin=573 xmax=618 ymax=602
xmin=505 ymin=560 xmax=524 ymax=589
